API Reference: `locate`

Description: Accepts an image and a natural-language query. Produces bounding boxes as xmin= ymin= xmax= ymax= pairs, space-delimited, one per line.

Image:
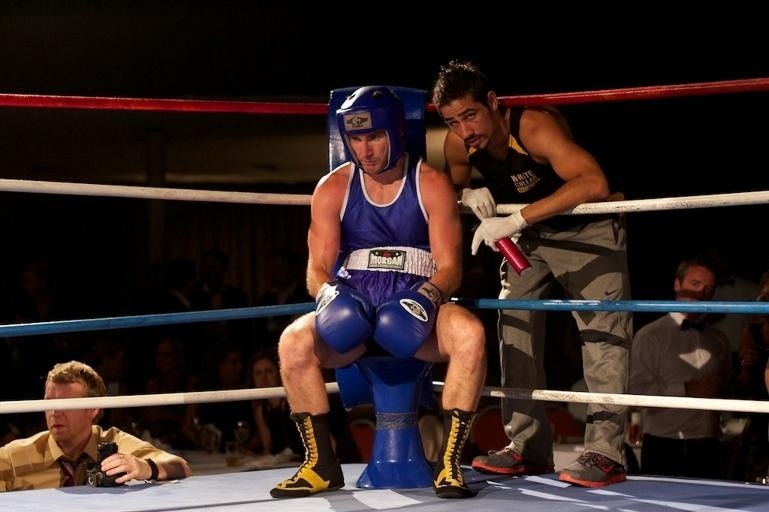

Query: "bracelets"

xmin=147 ymin=458 xmax=159 ymax=480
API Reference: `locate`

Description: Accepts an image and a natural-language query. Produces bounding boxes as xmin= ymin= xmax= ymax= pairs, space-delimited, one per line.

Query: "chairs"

xmin=351 ymin=400 xmax=640 ymax=478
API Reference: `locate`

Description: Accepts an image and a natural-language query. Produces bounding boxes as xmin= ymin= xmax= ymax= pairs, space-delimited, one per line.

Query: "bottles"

xmin=494 ymin=237 xmax=534 ymax=276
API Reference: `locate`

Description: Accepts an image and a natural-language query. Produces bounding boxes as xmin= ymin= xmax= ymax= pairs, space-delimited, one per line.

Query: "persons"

xmin=268 ymin=85 xmax=486 ymax=499
xmin=733 ymin=270 xmax=769 ymax=483
xmin=433 ymin=62 xmax=634 ymax=486
xmin=0 ymin=358 xmax=192 ymax=491
xmin=629 ymin=258 xmax=733 ymax=479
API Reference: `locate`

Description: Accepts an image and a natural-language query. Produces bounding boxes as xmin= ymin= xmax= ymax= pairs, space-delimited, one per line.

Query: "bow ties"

xmin=680 ymin=318 xmax=706 ymax=332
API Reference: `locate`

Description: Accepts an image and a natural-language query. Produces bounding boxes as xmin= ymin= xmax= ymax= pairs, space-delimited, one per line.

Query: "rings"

xmin=741 ymin=360 xmax=744 ymax=365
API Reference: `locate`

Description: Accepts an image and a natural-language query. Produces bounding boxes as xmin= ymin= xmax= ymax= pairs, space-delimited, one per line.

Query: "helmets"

xmin=336 ymin=83 xmax=410 ymax=176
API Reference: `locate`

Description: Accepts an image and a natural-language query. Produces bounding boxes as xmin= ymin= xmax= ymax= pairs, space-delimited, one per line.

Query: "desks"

xmin=180 ymin=450 xmax=302 ymax=476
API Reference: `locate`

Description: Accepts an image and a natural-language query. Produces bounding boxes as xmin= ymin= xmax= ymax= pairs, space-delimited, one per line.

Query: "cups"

xmin=224 ymin=423 xmax=248 ymax=468
xmin=624 ymin=422 xmax=641 ymax=448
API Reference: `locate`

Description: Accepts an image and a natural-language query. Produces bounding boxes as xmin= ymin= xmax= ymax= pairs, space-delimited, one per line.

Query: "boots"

xmin=431 ymin=411 xmax=479 ymax=501
xmin=268 ymin=409 xmax=347 ymax=499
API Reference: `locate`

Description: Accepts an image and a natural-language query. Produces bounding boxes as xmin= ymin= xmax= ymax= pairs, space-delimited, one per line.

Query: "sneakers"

xmin=558 ymin=449 xmax=627 ymax=490
xmin=470 ymin=440 xmax=557 ymax=477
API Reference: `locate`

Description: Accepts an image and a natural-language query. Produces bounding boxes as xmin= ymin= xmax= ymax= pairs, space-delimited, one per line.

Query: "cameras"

xmin=85 ymin=441 xmax=127 ymax=487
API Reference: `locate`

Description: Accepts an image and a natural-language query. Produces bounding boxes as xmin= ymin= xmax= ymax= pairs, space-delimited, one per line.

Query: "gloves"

xmin=468 ymin=207 xmax=532 ymax=257
xmin=459 ymin=186 xmax=499 ymax=223
xmin=372 ymin=279 xmax=445 ymax=362
xmin=314 ymin=281 xmax=374 ymax=355
